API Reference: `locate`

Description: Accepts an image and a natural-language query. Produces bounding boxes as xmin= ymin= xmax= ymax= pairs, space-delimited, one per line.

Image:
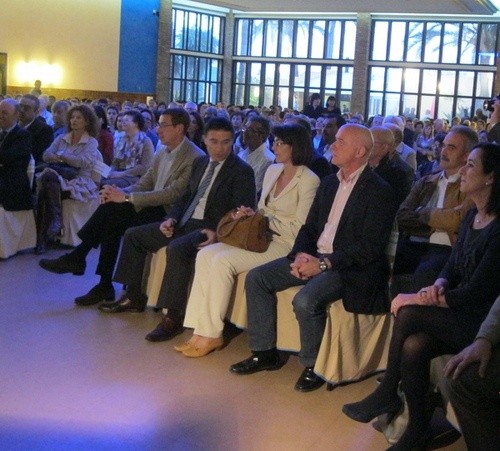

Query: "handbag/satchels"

xmin=216 ymin=209 xmax=270 ymax=253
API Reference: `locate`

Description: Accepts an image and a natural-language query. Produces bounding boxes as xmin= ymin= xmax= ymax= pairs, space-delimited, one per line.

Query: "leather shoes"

xmin=295 ymin=368 xmax=324 ymax=392
xmin=39 ymin=253 xmax=86 ymax=276
xmin=98 ymin=293 xmax=148 ymax=313
xmin=74 ymin=284 xmax=115 ymax=304
xmin=231 ymin=354 xmax=283 ymax=374
xmin=145 ymin=314 xmax=185 ymax=342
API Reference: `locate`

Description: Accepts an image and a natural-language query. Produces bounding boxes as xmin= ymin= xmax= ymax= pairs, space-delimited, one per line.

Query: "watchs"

xmin=123 ymin=194 xmax=130 ymax=202
xmin=319 ymin=257 xmax=328 ymax=272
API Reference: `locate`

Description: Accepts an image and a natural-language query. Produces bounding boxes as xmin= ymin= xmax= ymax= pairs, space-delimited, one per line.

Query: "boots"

xmin=36 ymin=191 xmax=50 ymax=254
xmin=46 ymin=180 xmax=65 ymax=238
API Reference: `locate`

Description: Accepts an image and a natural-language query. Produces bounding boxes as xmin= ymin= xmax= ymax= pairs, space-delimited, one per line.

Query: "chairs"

xmin=0 ymin=195 xmax=400 ymax=380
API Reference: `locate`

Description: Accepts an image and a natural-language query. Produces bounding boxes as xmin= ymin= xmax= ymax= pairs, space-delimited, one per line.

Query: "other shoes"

xmin=175 ymin=339 xmax=192 ymax=352
xmin=183 ymin=337 xmax=224 ymax=358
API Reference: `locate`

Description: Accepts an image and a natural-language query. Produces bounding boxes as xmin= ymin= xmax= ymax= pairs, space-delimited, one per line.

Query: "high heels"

xmin=342 ymin=395 xmax=402 ymax=424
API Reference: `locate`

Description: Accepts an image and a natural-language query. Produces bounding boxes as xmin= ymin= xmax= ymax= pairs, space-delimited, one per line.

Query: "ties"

xmin=177 ymin=161 xmax=219 ymax=229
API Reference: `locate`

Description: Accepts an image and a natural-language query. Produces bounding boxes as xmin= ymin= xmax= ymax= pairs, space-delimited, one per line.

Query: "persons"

xmin=230 ymin=124 xmax=396 ymax=392
xmin=98 ymin=116 xmax=256 ymax=344
xmin=0 ymin=90 xmax=500 ymax=294
xmin=302 ymin=93 xmax=325 ymax=119
xmin=323 ymin=96 xmax=341 ymax=115
xmin=0 ymin=99 xmax=34 ymax=212
xmin=38 ymin=108 xmax=206 ymax=306
xmin=342 ymin=144 xmax=500 ymax=451
xmin=31 ymin=80 xmax=42 ymax=95
xmin=442 ymin=295 xmax=500 ymax=451
xmin=172 ymin=123 xmax=320 ymax=358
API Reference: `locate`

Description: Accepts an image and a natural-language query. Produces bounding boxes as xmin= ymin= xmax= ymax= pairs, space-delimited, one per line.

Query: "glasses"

xmin=157 ymin=122 xmax=177 ymax=129
xmin=273 ymin=141 xmax=290 ymax=148
xmin=242 ymin=128 xmax=265 ymax=135
